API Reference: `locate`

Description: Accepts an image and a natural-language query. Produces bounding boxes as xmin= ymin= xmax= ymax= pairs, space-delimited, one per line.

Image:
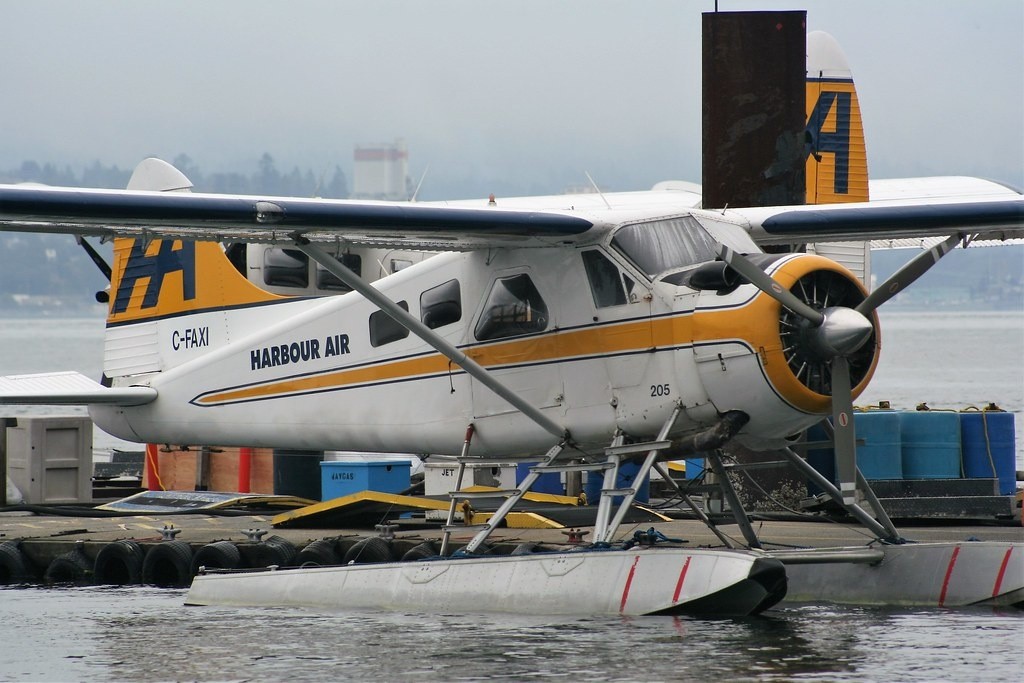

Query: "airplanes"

xmin=0 ymin=157 xmax=1024 ymax=456
